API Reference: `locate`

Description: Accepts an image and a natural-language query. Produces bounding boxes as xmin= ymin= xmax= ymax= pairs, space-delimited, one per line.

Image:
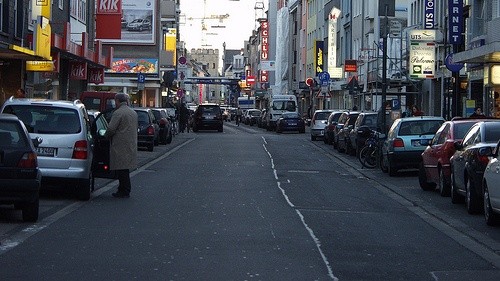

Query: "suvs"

xmin=309 ymin=109 xmax=394 ymax=156
xmin=0 ymin=96 xmax=95 ymax=202
xmin=418 ymin=117 xmax=500 ymax=196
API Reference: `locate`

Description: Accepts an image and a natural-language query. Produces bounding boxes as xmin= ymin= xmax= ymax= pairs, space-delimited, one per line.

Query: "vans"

xmin=81 ymin=92 xmax=130 ymax=124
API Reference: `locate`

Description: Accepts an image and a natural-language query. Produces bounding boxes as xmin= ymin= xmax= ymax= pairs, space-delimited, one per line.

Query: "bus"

xmin=237 ymin=96 xmax=256 ymax=121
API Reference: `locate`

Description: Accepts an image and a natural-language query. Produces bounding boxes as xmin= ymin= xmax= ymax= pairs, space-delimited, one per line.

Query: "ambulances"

xmin=266 ymin=95 xmax=298 ymax=130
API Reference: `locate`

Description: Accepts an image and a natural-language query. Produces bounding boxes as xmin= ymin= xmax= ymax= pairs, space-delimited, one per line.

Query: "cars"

xmin=449 ymin=121 xmax=500 ymax=215
xmin=482 ymin=140 xmax=500 ymax=225
xmin=276 ymin=112 xmax=305 ymax=135
xmin=244 ymin=109 xmax=266 ymax=128
xmin=0 ymin=114 xmax=43 ymax=222
xmin=131 ymin=104 xmax=178 ymax=151
xmin=220 ymin=107 xmax=236 ymax=121
xmin=52 ymin=110 xmax=109 ymax=163
xmin=192 ymin=104 xmax=224 ymax=133
xmin=381 ymin=116 xmax=450 ymax=176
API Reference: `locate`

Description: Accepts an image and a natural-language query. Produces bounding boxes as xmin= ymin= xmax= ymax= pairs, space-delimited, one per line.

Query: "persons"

xmin=103 ymin=94 xmax=138 ymax=197
xmin=385 ymin=102 xmax=392 ymax=109
xmin=411 ymin=106 xmax=424 ymax=116
xmin=352 ymin=104 xmax=357 ymax=111
xmin=400 ymin=104 xmax=412 ymax=118
xmin=470 ymin=104 xmax=490 ymax=119
xmin=179 ymin=102 xmax=193 ymax=133
xmin=7 ymin=88 xmax=33 ymax=133
xmin=235 ymin=107 xmax=242 ymax=127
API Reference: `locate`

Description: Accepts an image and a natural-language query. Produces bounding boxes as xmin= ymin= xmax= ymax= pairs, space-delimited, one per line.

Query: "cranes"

xmin=184 ymin=0 xmax=229 ymax=48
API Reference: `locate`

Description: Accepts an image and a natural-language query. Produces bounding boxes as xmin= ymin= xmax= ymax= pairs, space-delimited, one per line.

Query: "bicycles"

xmin=359 ymin=128 xmax=385 ymax=169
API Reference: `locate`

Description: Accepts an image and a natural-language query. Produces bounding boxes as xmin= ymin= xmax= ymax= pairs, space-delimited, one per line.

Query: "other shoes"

xmin=111 ymin=192 xmax=130 ymax=198
